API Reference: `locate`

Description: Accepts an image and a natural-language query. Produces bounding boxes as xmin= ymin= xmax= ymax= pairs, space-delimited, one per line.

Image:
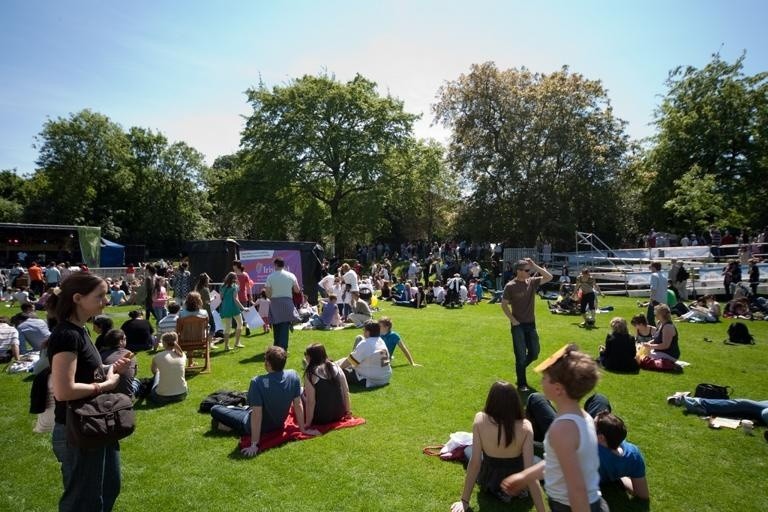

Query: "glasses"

xmin=519 ymin=269 xmax=531 ymax=272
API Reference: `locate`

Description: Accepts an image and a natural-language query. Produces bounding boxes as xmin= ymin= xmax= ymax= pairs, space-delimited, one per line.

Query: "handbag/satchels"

xmin=74 ymin=393 xmax=136 ymax=446
xmin=200 ymin=391 xmax=246 ymax=412
xmin=696 ymin=384 xmax=733 ymax=401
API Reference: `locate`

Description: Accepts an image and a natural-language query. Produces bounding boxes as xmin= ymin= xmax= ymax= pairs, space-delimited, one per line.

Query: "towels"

xmin=240 ymin=400 xmax=363 ymax=453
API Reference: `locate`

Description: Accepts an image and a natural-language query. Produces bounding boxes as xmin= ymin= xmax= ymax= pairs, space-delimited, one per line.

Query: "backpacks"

xmin=724 ymin=323 xmax=755 ymax=346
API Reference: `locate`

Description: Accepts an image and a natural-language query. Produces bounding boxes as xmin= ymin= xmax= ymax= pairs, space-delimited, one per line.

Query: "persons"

xmin=667 ymin=394 xmax=768 ymax=426
xmin=43 ymin=270 xmax=136 ymax=512
xmin=524 ymin=391 xmax=649 ymax=500
xmin=501 ymin=344 xmax=611 ymax=511
xmin=301 ymin=343 xmax=355 ymax=428
xmin=0 ymin=225 xmax=766 ymax=407
xmin=448 ymin=381 xmax=546 ymax=511
xmin=209 ymin=345 xmax=323 ymax=459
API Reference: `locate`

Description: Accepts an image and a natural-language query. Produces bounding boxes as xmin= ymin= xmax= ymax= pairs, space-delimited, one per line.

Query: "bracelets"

xmin=459 ymin=496 xmax=471 ymax=506
xmin=92 ymin=382 xmax=101 ymax=394
xmin=250 ymin=441 xmax=259 ymax=449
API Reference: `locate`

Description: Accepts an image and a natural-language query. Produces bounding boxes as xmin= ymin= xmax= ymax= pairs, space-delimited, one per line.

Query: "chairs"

xmin=174 ymin=316 xmax=212 ymax=374
xmin=359 ymin=287 xmax=371 ymax=307
